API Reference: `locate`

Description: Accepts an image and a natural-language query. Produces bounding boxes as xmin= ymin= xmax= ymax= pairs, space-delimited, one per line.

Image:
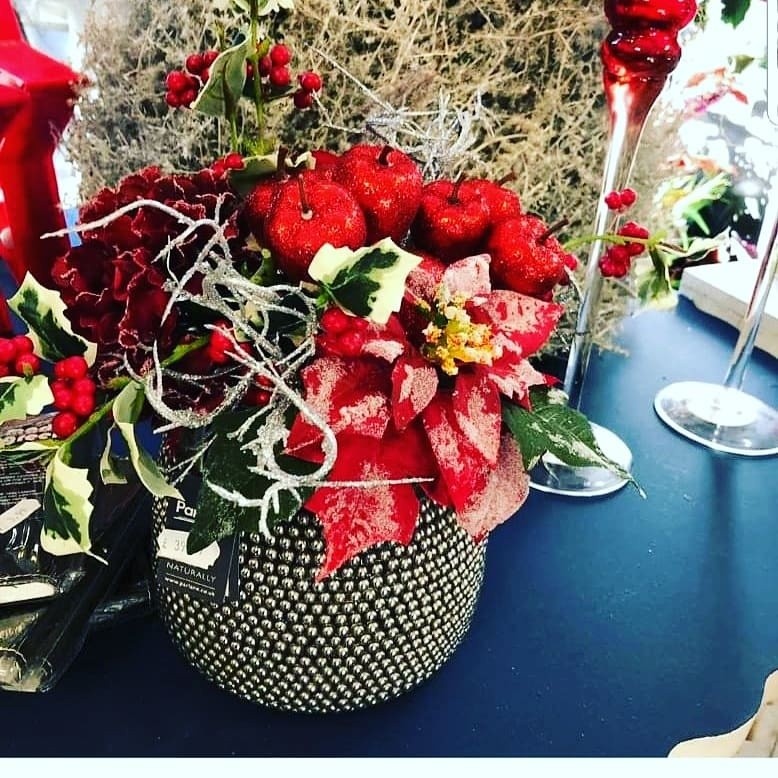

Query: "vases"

xmin=152 ymin=424 xmax=487 ymax=714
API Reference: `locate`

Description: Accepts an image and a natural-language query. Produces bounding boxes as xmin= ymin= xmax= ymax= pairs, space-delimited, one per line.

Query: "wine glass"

xmin=652 ymin=0 xmax=778 ymax=456
xmin=521 ymin=0 xmax=696 ymax=495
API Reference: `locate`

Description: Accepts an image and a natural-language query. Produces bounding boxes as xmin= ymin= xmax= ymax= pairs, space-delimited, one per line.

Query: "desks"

xmin=0 ymin=298 xmax=778 ymax=758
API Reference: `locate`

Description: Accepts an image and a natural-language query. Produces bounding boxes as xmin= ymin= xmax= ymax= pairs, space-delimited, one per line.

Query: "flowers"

xmin=0 ymin=0 xmax=649 ymax=565
